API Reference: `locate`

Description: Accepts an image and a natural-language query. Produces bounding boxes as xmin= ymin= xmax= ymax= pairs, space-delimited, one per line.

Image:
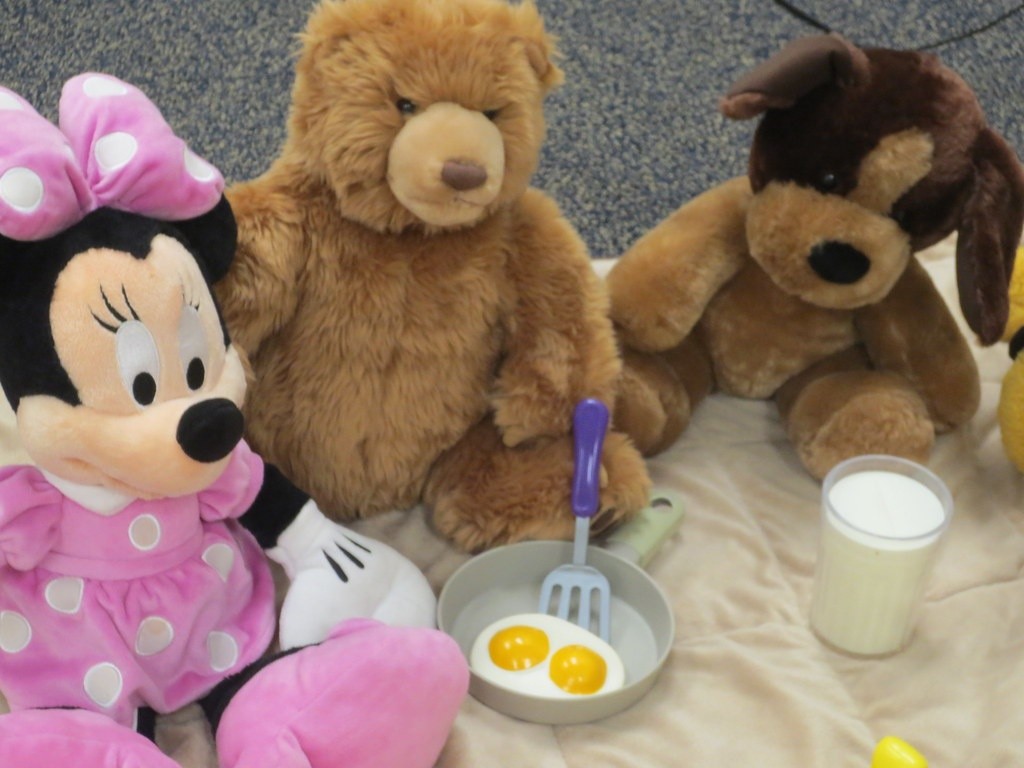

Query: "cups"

xmin=810 ymin=454 xmax=955 ymax=655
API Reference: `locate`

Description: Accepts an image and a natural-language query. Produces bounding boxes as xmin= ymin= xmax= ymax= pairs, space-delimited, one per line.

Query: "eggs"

xmin=470 ymin=613 xmax=625 ymax=700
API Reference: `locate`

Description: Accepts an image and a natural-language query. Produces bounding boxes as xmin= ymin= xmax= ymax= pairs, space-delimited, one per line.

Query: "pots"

xmin=437 ymin=491 xmax=686 ymax=725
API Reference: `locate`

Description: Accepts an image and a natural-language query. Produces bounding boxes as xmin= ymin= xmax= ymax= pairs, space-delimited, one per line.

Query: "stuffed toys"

xmin=604 ymin=32 xmax=1024 ymax=484
xmin=0 ymin=73 xmax=469 ymax=768
xmin=208 ymin=1 xmax=650 ymax=559
